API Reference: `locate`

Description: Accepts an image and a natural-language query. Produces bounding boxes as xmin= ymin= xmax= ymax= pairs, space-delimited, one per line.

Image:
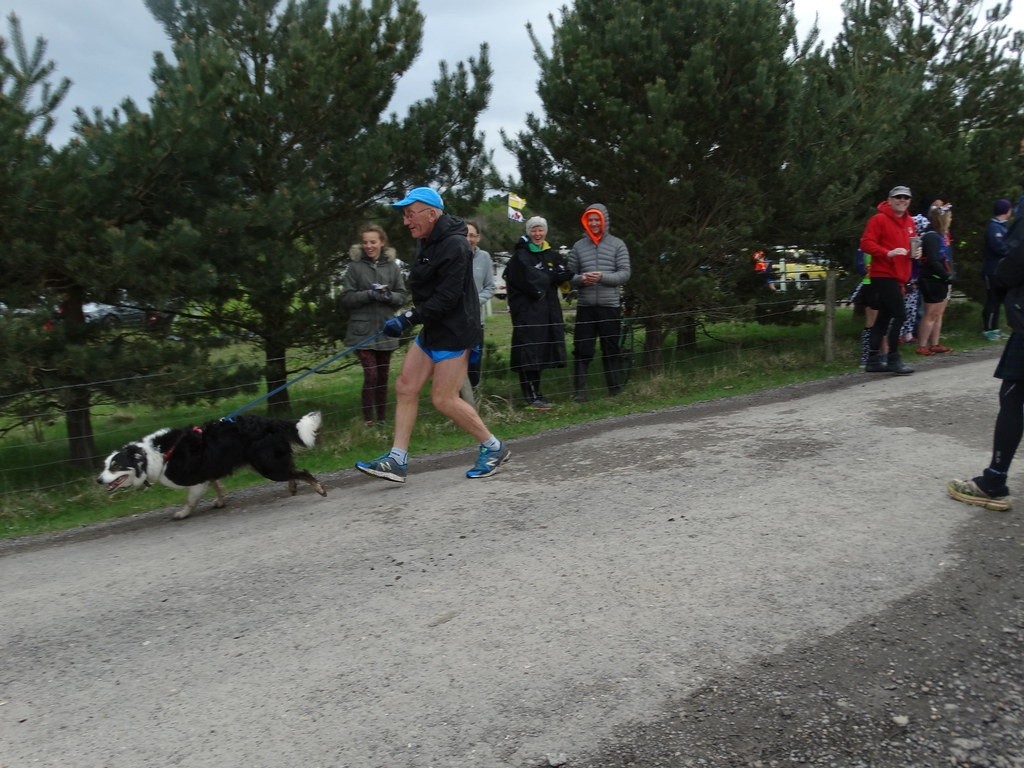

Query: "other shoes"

xmin=472 ymin=384 xmax=481 ymax=410
xmin=572 ymin=395 xmax=584 ymax=402
xmin=906 ymin=337 xmax=918 ymax=344
xmin=929 ymin=344 xmax=954 ymax=353
xmin=993 ymin=328 xmax=1011 ymax=339
xmin=916 ymin=346 xmax=935 ymax=356
xmin=524 ymin=399 xmax=552 ymax=410
xmin=983 ymin=330 xmax=1000 ymax=341
xmin=898 ymin=339 xmax=904 ymax=344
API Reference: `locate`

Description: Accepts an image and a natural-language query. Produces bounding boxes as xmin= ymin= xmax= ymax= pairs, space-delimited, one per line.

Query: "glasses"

xmin=401 ymin=208 xmax=431 ymax=220
xmin=896 ymin=195 xmax=909 ymax=200
xmin=467 ymin=233 xmax=477 ymax=238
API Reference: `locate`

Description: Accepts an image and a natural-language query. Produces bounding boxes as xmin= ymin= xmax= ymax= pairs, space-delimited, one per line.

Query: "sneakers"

xmin=946 ymin=468 xmax=1011 ymax=512
xmin=355 ymin=452 xmax=408 ymax=483
xmin=465 ymin=441 xmax=512 ymax=477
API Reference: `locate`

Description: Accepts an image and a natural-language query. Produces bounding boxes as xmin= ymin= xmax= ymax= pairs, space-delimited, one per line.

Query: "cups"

xmin=909 ymin=237 xmax=920 ymax=258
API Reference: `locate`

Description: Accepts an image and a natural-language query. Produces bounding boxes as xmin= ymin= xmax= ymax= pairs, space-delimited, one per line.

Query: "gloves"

xmin=552 ymin=269 xmax=575 ymax=286
xmin=372 ymin=284 xmax=392 ymax=304
xmin=383 ymin=315 xmax=410 ymax=337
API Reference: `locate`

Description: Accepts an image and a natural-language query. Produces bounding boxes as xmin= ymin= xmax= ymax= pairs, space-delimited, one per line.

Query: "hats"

xmin=526 ymin=216 xmax=548 ymax=238
xmin=391 ymin=187 xmax=444 ymax=210
xmin=993 ymin=199 xmax=1011 ymax=215
xmin=889 ymin=186 xmax=912 ymax=198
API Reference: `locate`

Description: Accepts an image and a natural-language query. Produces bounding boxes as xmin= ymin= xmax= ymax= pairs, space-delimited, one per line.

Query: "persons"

xmin=355 ymin=187 xmax=511 ymax=482
xmin=463 ymin=220 xmax=496 ymax=387
xmin=338 ymin=224 xmax=406 ymax=426
xmin=502 ymin=215 xmax=574 ymax=410
xmin=566 ymin=204 xmax=631 ymax=401
xmin=982 ymin=200 xmax=1012 ymax=340
xmin=898 ymin=200 xmax=954 ymax=355
xmin=847 ymin=249 xmax=889 ymax=368
xmin=860 ymin=186 xmax=922 ymax=373
xmin=947 ymin=199 xmax=1024 ymax=510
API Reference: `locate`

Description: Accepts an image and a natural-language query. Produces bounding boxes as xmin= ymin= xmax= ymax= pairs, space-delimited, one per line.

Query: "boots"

xmin=865 ymin=350 xmax=888 ymax=372
xmin=859 ymin=327 xmax=872 ymax=369
xmin=887 ymin=352 xmax=914 ymax=373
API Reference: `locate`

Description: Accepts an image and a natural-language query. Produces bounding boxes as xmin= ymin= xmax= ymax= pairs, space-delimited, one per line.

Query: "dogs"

xmin=95 ymin=409 xmax=327 ymax=521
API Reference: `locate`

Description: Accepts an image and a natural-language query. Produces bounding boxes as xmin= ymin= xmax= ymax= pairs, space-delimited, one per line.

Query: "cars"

xmin=733 ymin=247 xmax=832 ymax=287
xmin=489 ymin=256 xmax=510 ymax=300
xmin=52 ymin=286 xmax=149 ymax=332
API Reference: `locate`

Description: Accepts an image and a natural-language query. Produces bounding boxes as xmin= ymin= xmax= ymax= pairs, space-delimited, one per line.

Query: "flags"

xmin=508 ymin=192 xmax=527 ymax=209
xmin=508 ymin=207 xmax=523 ymax=222
